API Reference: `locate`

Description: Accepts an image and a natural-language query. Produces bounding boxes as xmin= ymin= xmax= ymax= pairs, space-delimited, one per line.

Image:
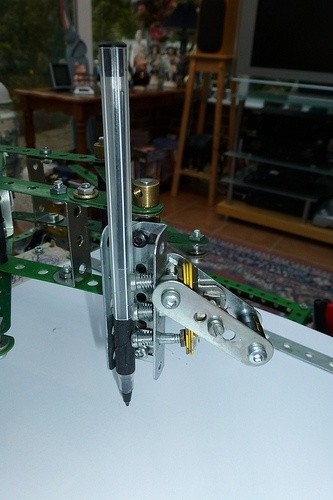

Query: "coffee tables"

xmin=215 ymin=72 xmax=333 ymax=250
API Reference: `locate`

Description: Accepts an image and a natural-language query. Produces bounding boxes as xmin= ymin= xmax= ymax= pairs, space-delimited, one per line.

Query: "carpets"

xmin=168 ymin=222 xmax=332 ymax=327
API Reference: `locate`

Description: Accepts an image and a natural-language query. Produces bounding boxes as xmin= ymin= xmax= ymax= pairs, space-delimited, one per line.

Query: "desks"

xmin=13 ymin=79 xmax=203 ymax=155
xmin=1 ymin=239 xmax=333 ymax=499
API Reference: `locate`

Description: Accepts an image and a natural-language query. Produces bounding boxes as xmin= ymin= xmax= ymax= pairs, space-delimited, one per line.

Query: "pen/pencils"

xmin=97 ymin=40 xmax=139 ymax=408
